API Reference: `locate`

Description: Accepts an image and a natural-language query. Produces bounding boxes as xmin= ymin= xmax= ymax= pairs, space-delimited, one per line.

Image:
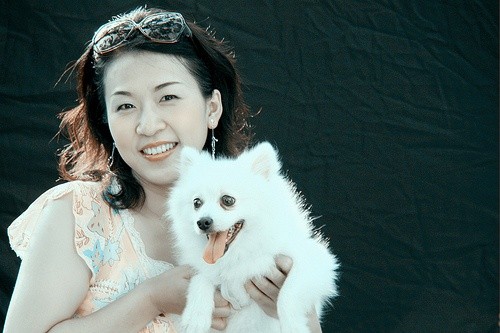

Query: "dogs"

xmin=163 ymin=140 xmax=338 ymax=333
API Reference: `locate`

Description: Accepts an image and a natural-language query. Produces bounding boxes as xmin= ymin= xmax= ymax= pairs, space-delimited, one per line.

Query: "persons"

xmin=1 ymin=7 xmax=322 ymax=333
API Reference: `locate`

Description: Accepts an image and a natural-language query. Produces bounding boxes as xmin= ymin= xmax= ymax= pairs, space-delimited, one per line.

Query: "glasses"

xmin=93 ymin=12 xmax=193 ymax=69
